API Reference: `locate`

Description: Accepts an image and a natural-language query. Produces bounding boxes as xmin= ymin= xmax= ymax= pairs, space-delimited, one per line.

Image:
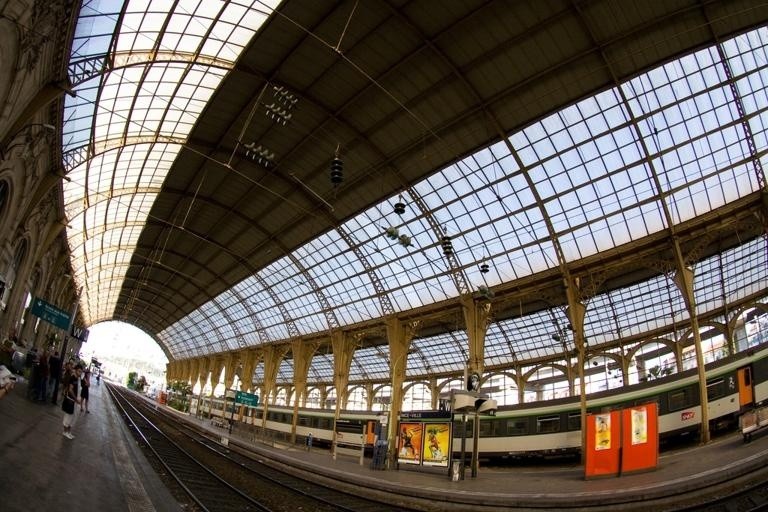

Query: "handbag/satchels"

xmin=62 ymin=396 xmax=75 ymax=414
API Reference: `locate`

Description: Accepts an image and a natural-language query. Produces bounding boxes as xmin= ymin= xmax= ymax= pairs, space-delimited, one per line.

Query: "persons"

xmin=307 ymin=433 xmax=312 ymax=452
xmin=80 ymin=372 xmax=90 ymax=413
xmin=62 ymin=355 xmax=80 ymax=396
xmin=0 ymin=381 xmax=16 ymax=400
xmin=33 ymin=351 xmax=49 ymax=402
xmin=23 ymin=339 xmax=27 ymax=348
xmin=47 ymin=351 xmax=62 ymax=405
xmin=62 ymin=363 xmax=83 ymax=440
xmin=97 ymin=374 xmax=100 ymax=385
xmin=401 ymin=427 xmax=415 ymax=455
xmin=429 ymin=433 xmax=440 ymax=458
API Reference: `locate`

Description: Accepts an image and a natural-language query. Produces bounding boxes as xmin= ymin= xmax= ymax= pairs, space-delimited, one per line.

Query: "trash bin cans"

xmin=586 ymin=399 xmax=658 ymax=480
xmin=450 ymin=458 xmax=465 ymax=482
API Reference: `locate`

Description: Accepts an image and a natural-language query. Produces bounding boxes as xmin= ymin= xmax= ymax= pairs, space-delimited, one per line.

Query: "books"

xmin=0 ymin=365 xmax=18 ymax=388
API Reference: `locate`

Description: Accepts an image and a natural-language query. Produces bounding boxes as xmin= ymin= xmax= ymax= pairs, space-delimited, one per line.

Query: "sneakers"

xmin=80 ymin=409 xmax=90 ymax=414
xmin=62 ymin=431 xmax=75 ymax=439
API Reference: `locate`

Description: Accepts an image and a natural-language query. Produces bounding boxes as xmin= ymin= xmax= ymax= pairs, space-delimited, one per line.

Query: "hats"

xmin=73 ymin=364 xmax=83 ymax=370
